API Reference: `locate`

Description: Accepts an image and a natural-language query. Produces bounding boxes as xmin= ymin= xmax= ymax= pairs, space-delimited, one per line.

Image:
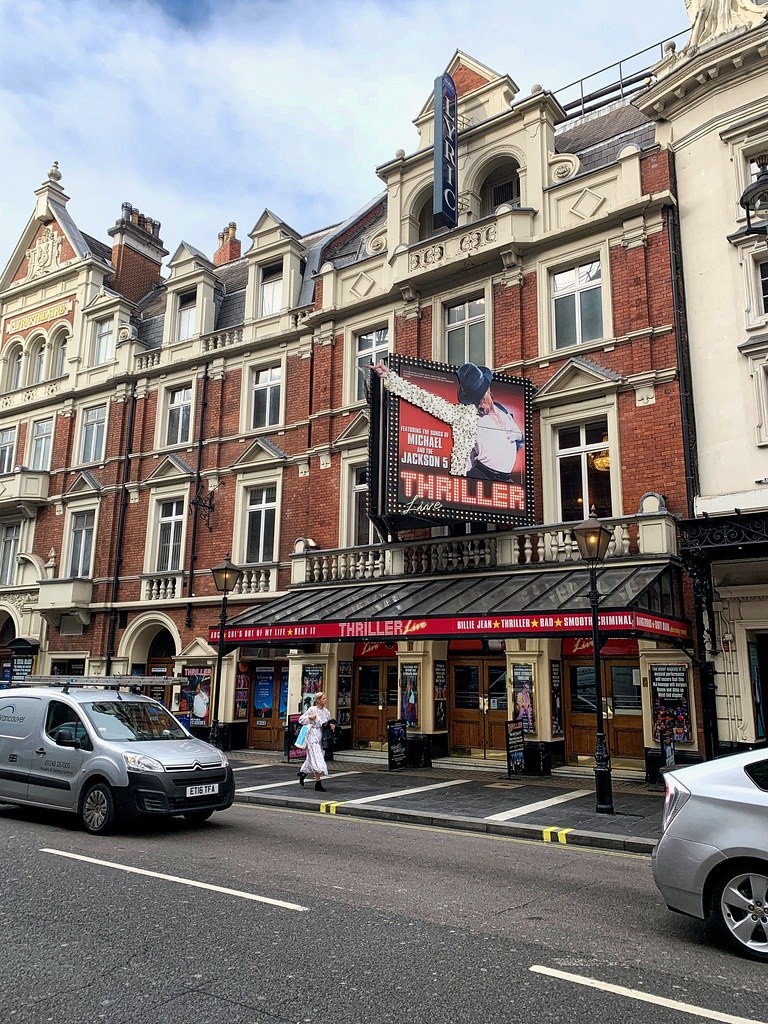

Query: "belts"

xmin=474 ymin=460 xmax=511 ymax=480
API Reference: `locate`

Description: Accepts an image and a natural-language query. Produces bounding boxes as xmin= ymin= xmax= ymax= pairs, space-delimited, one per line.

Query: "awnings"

xmin=208 ymin=560 xmax=693 ymax=647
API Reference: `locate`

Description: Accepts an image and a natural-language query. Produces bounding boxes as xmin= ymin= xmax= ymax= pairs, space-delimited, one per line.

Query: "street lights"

xmin=568 ymin=503 xmax=616 ymax=813
xmin=208 ymin=549 xmax=244 ymax=744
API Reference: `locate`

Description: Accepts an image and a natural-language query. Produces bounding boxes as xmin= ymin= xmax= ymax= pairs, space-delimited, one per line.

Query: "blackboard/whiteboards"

xmin=659 ymin=725 xmax=676 ymax=766
xmin=386 ymin=718 xmax=409 ymax=770
xmin=503 ymin=718 xmax=527 ymax=775
xmin=288 ymin=714 xmax=307 ymax=759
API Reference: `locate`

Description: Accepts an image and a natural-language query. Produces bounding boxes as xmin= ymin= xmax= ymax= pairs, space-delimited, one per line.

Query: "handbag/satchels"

xmin=294 ymin=725 xmax=311 ymax=749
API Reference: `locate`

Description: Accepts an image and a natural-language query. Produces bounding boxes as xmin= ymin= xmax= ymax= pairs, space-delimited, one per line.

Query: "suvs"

xmin=0 ymin=673 xmax=237 ymax=836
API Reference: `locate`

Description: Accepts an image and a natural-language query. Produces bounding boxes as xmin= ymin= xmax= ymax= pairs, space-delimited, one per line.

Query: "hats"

xmin=522 ymin=684 xmax=530 ymax=690
xmin=315 ymin=692 xmax=324 ymax=700
xmin=456 ymin=363 xmax=493 ymax=404
xmin=304 ymin=697 xmax=311 ymax=705
xmin=187 ymin=674 xmax=202 ymax=691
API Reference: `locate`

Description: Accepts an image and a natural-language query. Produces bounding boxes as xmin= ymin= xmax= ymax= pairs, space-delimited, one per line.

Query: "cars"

xmin=651 ymin=746 xmax=768 ymax=963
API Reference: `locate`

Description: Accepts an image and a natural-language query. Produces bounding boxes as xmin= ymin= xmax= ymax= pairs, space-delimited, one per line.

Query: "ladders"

xmin=11 ymin=674 xmax=191 ymax=686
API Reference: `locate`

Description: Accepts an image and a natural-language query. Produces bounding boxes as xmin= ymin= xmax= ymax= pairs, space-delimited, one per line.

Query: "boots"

xmin=315 ymin=780 xmax=326 ymax=791
xmin=297 ymin=772 xmax=307 ymax=786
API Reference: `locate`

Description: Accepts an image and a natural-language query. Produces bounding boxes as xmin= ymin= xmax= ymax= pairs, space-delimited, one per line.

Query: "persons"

xmin=294 ymin=692 xmax=335 ymax=792
xmin=364 ymin=363 xmax=522 ymax=483
xmin=403 ymin=683 xmax=419 ymax=726
xmin=192 ymin=682 xmax=209 ymax=720
xmin=516 ymin=684 xmax=533 ymax=728
